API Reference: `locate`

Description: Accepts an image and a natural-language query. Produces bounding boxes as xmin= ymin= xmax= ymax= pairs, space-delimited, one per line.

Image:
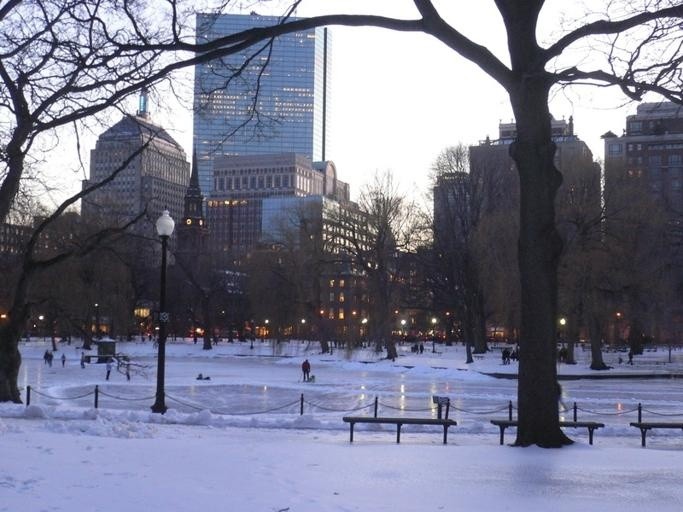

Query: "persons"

xmin=48 ymin=352 xmax=52 ymax=368
xmin=80 ymin=351 xmax=85 ymax=368
xmin=104 ymin=361 xmax=111 ymax=379
xmin=419 ymin=342 xmax=424 ymax=353
xmin=415 ymin=344 xmax=418 ymax=354
xmin=43 ymin=351 xmax=48 ymax=363
xmin=557 ymin=383 xmax=567 ymax=410
xmin=301 ymin=359 xmax=310 ymax=380
xmin=627 ymin=350 xmax=633 ymax=365
xmin=60 ymin=353 xmax=65 ymax=365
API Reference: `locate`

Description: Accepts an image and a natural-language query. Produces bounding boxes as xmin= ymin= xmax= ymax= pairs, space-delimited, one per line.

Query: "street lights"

xmin=153 ymin=209 xmax=175 ymax=416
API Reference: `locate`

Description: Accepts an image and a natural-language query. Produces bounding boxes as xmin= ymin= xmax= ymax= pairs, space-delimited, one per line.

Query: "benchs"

xmin=631 ymin=420 xmax=681 ymax=445
xmin=343 ymin=415 xmax=459 ymax=448
xmin=487 ymin=418 xmax=605 ymax=447
